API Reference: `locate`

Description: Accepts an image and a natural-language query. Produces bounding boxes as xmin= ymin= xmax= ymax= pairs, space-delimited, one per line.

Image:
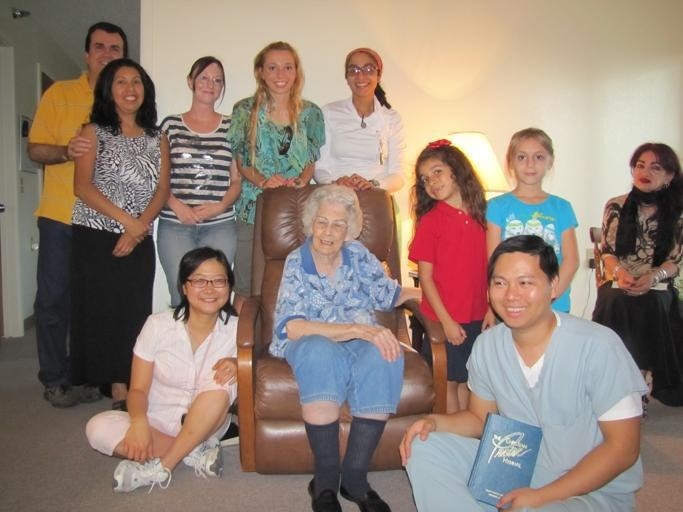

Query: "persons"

xmin=71 ymin=59 xmax=171 ymax=411
xmin=156 ymin=57 xmax=243 ymax=309
xmin=268 ymin=185 xmax=422 ymax=512
xmin=26 ymin=22 xmax=128 ymax=408
xmin=480 ymin=128 xmax=580 ymax=332
xmin=408 ymin=139 xmax=496 ymax=412
xmin=311 ymin=48 xmax=405 ymax=194
xmin=84 ymin=247 xmax=239 ymax=494
xmin=397 ymin=234 xmax=649 ymax=511
xmin=227 ymin=42 xmax=325 ymax=315
xmin=593 ymin=143 xmax=683 ymax=407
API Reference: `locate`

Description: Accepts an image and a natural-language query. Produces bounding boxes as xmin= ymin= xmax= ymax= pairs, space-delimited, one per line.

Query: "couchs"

xmin=236 ymin=182 xmax=448 ymax=475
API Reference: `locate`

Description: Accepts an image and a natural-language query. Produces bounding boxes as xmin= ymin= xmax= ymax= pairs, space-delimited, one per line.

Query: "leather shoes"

xmin=307 ymin=479 xmax=341 ymax=511
xmin=339 ymin=485 xmax=390 ymax=512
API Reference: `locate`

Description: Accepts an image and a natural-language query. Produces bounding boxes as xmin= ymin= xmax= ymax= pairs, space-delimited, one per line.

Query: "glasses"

xmin=279 ymin=125 xmax=292 ymax=154
xmin=188 ymin=278 xmax=228 ymax=288
xmin=349 ymin=66 xmax=375 ymax=74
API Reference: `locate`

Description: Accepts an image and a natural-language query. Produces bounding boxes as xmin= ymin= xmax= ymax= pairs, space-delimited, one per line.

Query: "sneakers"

xmin=44 ymin=385 xmax=127 ymax=411
xmin=112 ymin=401 xmax=128 ymax=412
xmin=112 ymin=456 xmax=171 ymax=493
xmin=183 ymin=435 xmax=223 ymax=480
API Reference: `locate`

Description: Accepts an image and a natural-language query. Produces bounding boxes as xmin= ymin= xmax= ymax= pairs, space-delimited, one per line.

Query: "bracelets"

xmin=612 ymin=265 xmax=622 ymax=280
xmin=654 ymin=268 xmax=667 ymax=283
xmin=368 ymin=179 xmax=380 ymax=187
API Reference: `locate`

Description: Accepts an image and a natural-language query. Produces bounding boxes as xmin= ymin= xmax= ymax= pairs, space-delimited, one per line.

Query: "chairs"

xmin=589 ymin=227 xmax=602 ymax=285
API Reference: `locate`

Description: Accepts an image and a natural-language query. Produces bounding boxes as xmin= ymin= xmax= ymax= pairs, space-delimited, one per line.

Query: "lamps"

xmin=446 ymin=132 xmax=511 ymax=200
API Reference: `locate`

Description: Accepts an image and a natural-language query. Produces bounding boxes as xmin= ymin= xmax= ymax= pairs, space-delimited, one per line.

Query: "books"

xmin=467 ymin=412 xmax=543 ymax=510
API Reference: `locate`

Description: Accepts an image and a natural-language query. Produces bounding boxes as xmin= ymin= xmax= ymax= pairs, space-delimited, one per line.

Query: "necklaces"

xmin=356 ymin=106 xmax=371 ymax=129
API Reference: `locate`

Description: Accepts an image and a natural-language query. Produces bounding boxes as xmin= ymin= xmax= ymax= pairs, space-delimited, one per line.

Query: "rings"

xmin=639 ymin=291 xmax=642 ymax=296
